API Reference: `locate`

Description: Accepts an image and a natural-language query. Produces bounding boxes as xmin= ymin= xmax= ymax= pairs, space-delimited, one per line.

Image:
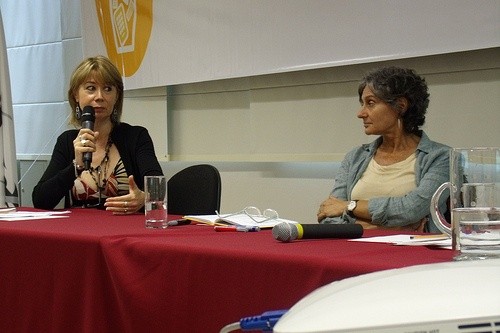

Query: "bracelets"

xmin=73 ymin=159 xmax=83 ymax=177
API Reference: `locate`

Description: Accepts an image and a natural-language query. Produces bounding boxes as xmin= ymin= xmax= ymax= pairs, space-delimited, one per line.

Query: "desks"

xmin=0 ymin=206 xmax=459 ymax=333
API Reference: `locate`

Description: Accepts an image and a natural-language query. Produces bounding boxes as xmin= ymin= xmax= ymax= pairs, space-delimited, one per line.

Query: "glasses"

xmin=213 ymin=205 xmax=278 ymax=224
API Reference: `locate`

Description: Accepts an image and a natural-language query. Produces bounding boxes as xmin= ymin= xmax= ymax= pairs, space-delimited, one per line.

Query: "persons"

xmin=32 ymin=55 xmax=166 ymax=215
xmin=316 ymin=67 xmax=464 ymax=234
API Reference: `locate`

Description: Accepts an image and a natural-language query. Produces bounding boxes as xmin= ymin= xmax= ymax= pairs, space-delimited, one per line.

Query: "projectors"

xmin=271 ymin=258 xmax=500 ymax=333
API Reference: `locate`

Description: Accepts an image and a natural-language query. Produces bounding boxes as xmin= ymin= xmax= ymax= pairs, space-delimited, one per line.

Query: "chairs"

xmin=167 ymin=165 xmax=221 ymax=215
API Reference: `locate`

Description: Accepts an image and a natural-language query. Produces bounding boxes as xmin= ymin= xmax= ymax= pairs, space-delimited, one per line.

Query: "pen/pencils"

xmin=410 ymin=233 xmax=448 ymax=239
xmin=168 ymin=219 xmax=203 ymax=225
xmin=213 ymin=225 xmax=260 ymax=232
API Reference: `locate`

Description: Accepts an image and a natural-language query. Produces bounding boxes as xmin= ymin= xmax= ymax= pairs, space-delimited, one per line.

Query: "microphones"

xmin=272 ymin=222 xmax=364 ymax=243
xmin=81 ymin=105 xmax=96 ymax=170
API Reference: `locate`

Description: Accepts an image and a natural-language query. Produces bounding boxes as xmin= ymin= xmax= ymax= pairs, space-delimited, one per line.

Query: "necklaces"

xmin=84 ymin=142 xmax=110 ymax=187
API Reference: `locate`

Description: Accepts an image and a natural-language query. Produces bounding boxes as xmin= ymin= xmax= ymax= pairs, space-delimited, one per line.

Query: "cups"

xmin=429 ymin=147 xmax=500 ymax=261
xmin=144 ymin=175 xmax=169 ymax=230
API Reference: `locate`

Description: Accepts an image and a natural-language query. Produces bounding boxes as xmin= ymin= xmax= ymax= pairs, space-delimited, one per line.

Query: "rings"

xmin=125 ymin=208 xmax=128 ymax=212
xmin=124 ymin=201 xmax=128 ymax=207
xmin=81 ymin=140 xmax=86 ymax=147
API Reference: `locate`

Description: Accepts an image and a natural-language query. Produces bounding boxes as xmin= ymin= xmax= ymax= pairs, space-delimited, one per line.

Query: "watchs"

xmin=347 ymin=200 xmax=359 ymax=217
